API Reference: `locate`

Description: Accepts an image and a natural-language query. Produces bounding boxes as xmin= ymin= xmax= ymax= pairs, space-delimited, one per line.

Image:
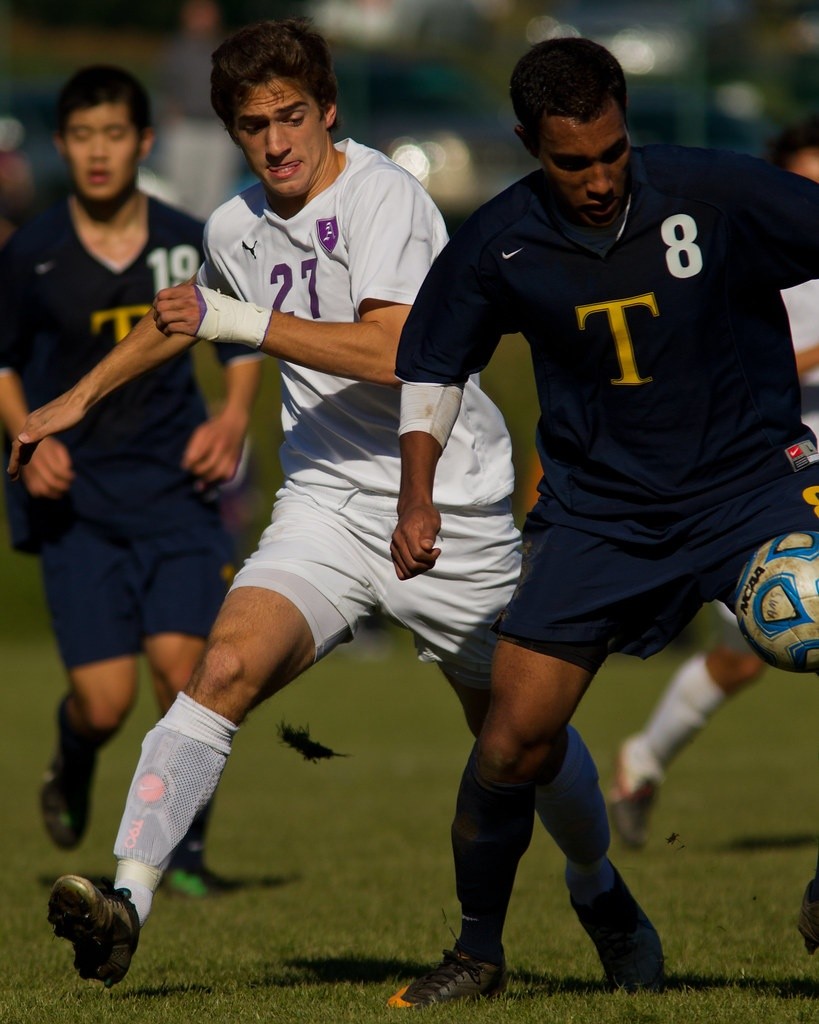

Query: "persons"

xmin=0 ymin=20 xmax=819 ymax=1010
xmin=389 ymin=39 xmax=819 ymax=1010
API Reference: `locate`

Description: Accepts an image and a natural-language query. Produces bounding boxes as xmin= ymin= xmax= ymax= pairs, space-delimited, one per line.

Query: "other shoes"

xmin=162 ymin=863 xmax=207 ymax=900
xmin=42 ymin=754 xmax=95 ymax=849
xmin=613 ymin=736 xmax=662 ymax=848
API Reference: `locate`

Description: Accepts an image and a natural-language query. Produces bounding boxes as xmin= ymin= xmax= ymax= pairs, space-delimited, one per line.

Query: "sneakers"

xmin=386 ymin=938 xmax=507 ymax=1011
xmin=569 ymin=860 xmax=663 ymax=994
xmin=47 ymin=875 xmax=140 ymax=988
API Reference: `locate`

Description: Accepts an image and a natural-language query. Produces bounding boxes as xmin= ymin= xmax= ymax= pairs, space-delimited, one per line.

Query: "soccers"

xmin=734 ymin=531 xmax=819 ymax=674
